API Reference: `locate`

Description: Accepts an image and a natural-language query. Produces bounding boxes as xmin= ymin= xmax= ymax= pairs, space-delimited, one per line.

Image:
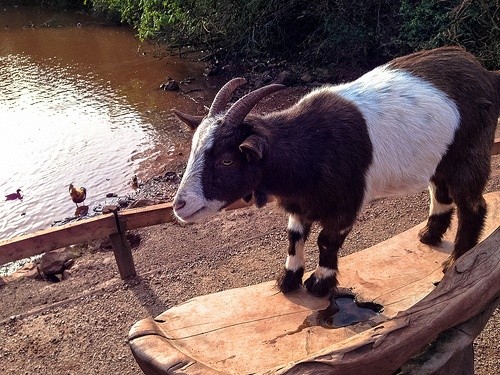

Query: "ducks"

xmin=5 ymin=189 xmax=23 ymax=200
xmin=69 ymin=184 xmax=87 ymax=210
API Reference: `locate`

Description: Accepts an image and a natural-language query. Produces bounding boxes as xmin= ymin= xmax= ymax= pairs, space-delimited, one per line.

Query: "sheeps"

xmin=168 ymin=46 xmax=500 ymax=298
